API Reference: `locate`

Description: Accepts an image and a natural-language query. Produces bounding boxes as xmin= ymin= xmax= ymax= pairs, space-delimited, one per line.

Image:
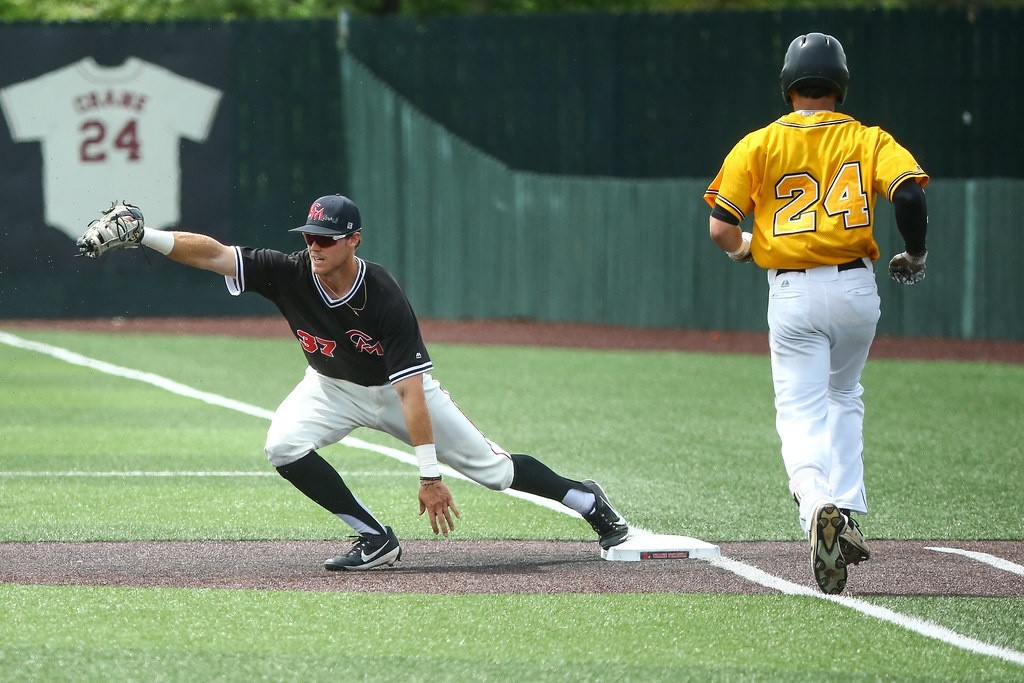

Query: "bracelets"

xmin=412 ymin=443 xmax=442 ymax=491
xmin=140 ymin=224 xmax=175 ymax=255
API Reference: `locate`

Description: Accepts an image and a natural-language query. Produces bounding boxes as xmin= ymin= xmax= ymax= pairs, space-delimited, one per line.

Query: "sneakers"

xmin=579 ymin=479 xmax=629 ymax=550
xmin=322 ymin=526 xmax=402 ymax=572
xmin=810 ymin=503 xmax=848 ymax=595
xmin=839 ymin=517 xmax=870 ymax=566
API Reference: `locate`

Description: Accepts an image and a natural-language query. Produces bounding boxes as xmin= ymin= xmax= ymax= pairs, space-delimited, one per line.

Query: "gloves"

xmin=888 ymin=251 xmax=927 ymax=286
xmin=725 ymin=231 xmax=753 ymax=263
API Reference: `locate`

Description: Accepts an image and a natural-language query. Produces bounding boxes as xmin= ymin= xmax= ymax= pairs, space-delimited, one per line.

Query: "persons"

xmin=72 ymin=194 xmax=631 ymax=573
xmin=702 ymin=31 xmax=931 ymax=596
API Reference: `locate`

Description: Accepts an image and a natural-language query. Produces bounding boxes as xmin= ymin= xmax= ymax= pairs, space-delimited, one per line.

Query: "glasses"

xmin=302 ymin=228 xmax=363 ymax=248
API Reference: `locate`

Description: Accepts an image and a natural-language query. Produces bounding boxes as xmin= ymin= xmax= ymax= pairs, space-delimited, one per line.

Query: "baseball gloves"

xmin=75 ymin=205 xmax=145 ymax=261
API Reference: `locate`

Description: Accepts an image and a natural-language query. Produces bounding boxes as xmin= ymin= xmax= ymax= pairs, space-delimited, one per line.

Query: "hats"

xmin=287 ymin=193 xmax=362 ymax=237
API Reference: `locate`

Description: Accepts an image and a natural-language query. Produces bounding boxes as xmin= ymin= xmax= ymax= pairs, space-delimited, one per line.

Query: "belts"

xmin=775 ymin=257 xmax=867 ymax=277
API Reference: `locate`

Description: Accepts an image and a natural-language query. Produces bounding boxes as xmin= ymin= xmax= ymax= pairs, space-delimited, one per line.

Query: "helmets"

xmin=778 ymin=33 xmax=850 ymax=106
